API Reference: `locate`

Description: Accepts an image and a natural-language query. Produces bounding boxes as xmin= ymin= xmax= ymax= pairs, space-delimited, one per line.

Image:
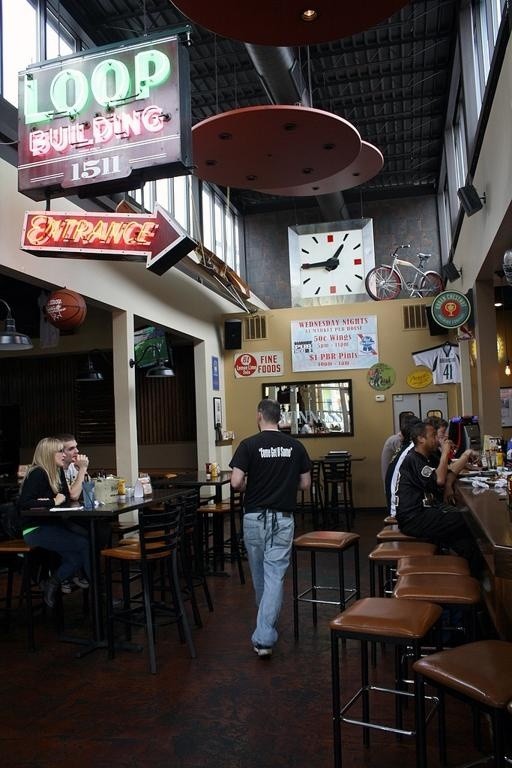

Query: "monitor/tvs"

xmin=447 ymin=418 xmax=481 ymax=459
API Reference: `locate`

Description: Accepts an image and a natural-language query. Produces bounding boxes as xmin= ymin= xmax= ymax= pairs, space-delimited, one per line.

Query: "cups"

xmin=81 ymin=482 xmax=95 ymax=508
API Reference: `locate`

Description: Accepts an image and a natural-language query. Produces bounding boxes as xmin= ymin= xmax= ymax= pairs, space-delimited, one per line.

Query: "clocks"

xmin=288 ymin=217 xmax=377 ymax=309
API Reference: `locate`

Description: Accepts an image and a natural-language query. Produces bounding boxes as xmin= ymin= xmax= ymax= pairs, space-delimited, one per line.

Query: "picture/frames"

xmin=499 ymin=386 xmax=512 ymax=428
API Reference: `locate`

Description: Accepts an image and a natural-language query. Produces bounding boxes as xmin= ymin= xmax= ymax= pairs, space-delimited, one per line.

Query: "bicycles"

xmin=365 ymin=245 xmax=444 ymax=302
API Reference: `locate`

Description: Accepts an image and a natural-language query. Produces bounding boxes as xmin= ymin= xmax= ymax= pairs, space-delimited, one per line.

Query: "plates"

xmin=459 ymin=477 xmax=487 ymax=484
xmin=460 ymin=471 xmax=481 ymax=476
xmin=489 ymin=479 xmax=505 ymax=485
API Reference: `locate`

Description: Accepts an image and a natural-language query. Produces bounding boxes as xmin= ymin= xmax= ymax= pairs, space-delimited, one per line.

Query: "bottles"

xmin=495 ymin=445 xmax=503 ymax=466
xmin=118 ymin=479 xmax=126 ymax=506
xmin=212 ymin=463 xmax=217 ymax=477
xmin=205 ymin=462 xmax=212 ymax=481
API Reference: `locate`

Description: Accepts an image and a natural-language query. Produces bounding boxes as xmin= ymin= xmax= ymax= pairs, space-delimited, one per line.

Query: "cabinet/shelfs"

xmin=442 ymin=450 xmax=512 ymax=642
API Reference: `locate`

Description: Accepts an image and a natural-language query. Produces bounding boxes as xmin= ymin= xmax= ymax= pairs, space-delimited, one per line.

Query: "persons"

xmin=381 ymin=411 xmax=415 ymax=494
xmin=394 ymin=422 xmax=474 ymax=555
xmin=385 ymin=415 xmax=423 ymax=516
xmin=229 ymin=399 xmax=314 ymax=658
xmin=390 ymin=416 xmax=476 ymax=517
xmin=301 ymin=419 xmax=313 ymax=434
xmin=57 ymin=433 xmax=91 ymax=594
xmin=17 ymin=437 xmax=88 ymax=608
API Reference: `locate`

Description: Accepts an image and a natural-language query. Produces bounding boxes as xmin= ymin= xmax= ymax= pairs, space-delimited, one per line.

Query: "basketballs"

xmin=44 ymin=289 xmax=88 ymax=330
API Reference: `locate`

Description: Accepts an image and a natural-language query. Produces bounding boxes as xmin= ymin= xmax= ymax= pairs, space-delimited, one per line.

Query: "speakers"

xmin=223 ymin=319 xmax=243 ymax=351
xmin=442 ymin=262 xmax=461 ymax=284
xmin=424 ymin=306 xmax=448 ymax=336
xmin=456 ymin=185 xmax=483 ymax=218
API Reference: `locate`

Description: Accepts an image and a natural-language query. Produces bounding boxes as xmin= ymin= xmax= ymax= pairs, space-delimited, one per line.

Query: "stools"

xmin=383 ymin=516 xmax=398 ymax=526
xmin=395 ymin=556 xmax=471 ymax=645
xmin=330 ymin=597 xmax=443 ymax=764
xmin=392 ymin=574 xmax=482 ymax=750
xmin=376 ymin=524 xmax=437 ymax=598
xmin=367 ymin=541 xmax=438 ymax=669
xmin=292 ymin=530 xmax=361 ymax=650
xmin=413 ymin=639 xmax=512 ymax=768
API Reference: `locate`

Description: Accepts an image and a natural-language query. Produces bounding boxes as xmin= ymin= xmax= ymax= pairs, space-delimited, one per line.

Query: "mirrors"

xmin=262 ymin=379 xmax=354 ymax=438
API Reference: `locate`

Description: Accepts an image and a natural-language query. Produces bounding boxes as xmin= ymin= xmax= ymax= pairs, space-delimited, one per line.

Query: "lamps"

xmin=73 ymin=347 xmax=113 ymax=382
xmin=0 ymin=298 xmax=34 ymax=351
xmin=130 ymin=345 xmax=175 ymax=378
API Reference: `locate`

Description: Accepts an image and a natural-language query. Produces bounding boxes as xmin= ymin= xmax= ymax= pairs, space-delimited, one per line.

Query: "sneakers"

xmin=74 ymin=577 xmax=89 ymax=588
xmin=253 ymin=644 xmax=272 ymax=657
xmin=61 ymin=580 xmax=72 ymax=594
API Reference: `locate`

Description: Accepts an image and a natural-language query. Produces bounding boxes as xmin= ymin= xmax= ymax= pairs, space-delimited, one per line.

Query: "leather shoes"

xmin=38 ymin=578 xmax=56 ymax=609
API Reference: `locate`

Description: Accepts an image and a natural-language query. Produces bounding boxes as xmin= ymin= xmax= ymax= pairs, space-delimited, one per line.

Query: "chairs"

xmin=296 ymin=460 xmax=325 ymax=531
xmin=218 ymin=489 xmax=246 ymax=564
xmin=0 ymin=504 xmax=63 ymax=653
xmin=99 ymin=506 xmax=197 ymax=674
xmin=196 ymin=478 xmax=247 ymax=584
xmin=118 ymin=502 xmax=203 ymax=644
xmin=148 ymin=495 xmax=214 ymax=625
xmin=321 ymin=461 xmax=355 ymax=531
